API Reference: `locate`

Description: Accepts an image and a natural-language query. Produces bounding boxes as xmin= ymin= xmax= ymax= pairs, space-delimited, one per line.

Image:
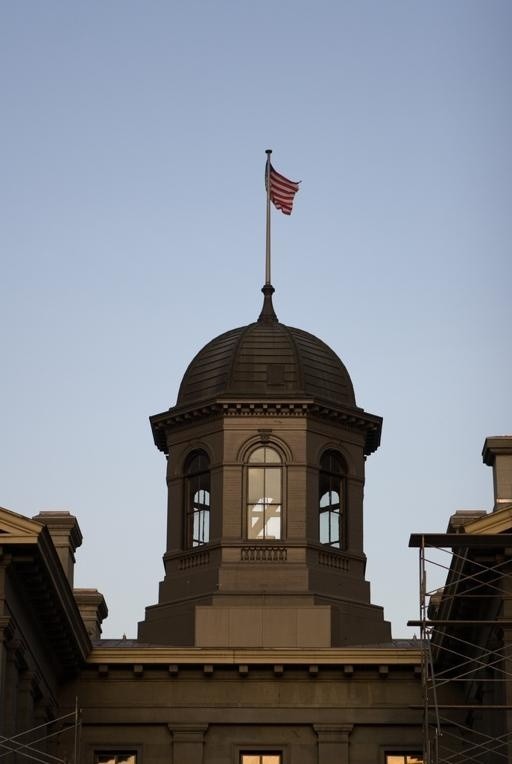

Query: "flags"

xmin=264 ymin=160 xmax=302 ymax=215
